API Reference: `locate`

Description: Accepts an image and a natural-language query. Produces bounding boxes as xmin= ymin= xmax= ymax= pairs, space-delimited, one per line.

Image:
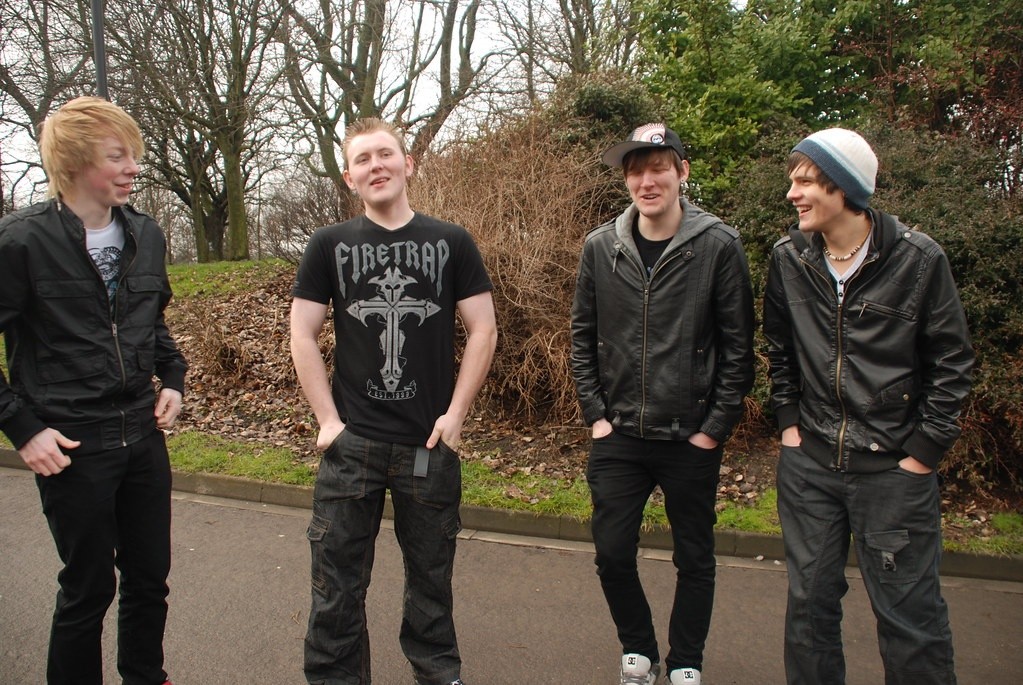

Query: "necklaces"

xmin=821 ymin=217 xmax=872 ymax=260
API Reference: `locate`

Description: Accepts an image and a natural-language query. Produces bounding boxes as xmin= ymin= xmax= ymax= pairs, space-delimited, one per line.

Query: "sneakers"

xmin=619 ymin=653 xmax=661 ymax=685
xmin=663 ymin=668 xmax=701 ymax=685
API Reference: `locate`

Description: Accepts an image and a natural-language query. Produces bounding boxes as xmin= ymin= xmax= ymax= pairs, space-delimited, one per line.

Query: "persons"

xmin=567 ymin=123 xmax=753 ymax=685
xmin=763 ymin=128 xmax=975 ymax=685
xmin=0 ymin=96 xmax=189 ymax=685
xmin=290 ymin=117 xmax=497 ymax=685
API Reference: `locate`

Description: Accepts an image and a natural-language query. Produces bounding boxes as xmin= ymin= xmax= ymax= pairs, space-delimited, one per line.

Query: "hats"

xmin=602 ymin=123 xmax=683 ymax=171
xmin=790 ymin=128 xmax=878 ymax=210
